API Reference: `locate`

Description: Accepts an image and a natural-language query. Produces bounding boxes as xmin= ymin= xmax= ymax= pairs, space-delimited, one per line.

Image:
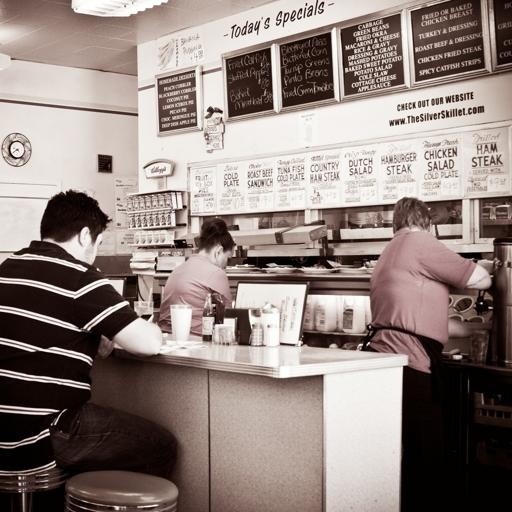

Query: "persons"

xmin=157 ymin=218 xmax=237 ymax=334
xmin=0 ymin=188 xmax=177 ymax=482
xmin=364 ymin=197 xmax=496 ymax=512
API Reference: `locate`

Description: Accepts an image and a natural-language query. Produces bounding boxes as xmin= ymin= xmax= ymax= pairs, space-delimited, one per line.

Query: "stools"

xmin=0 ymin=462 xmax=181 ymax=512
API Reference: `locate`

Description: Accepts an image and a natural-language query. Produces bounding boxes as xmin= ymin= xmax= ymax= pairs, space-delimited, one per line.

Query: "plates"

xmin=224 ymin=267 xmax=374 ymax=273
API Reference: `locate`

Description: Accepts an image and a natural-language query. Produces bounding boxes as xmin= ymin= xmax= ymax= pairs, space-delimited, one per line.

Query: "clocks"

xmin=9 ymin=140 xmax=26 ymax=158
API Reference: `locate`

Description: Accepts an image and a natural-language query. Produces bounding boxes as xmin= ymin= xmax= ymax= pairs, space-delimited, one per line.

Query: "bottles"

xmin=250 ymin=308 xmax=281 ymax=346
xmin=201 ymin=293 xmax=214 ymax=341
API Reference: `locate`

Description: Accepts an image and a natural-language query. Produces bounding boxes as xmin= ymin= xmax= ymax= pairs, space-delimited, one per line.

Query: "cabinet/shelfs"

xmin=128 ymin=189 xmax=189 ymax=229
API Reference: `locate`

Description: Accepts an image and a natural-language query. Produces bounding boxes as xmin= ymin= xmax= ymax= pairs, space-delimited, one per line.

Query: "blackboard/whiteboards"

xmin=221 ymin=23 xmax=340 ymax=123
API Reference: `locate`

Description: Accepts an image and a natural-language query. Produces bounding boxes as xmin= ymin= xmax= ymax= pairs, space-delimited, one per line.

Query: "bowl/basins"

xmin=355 ymin=212 xmax=377 ymax=224
xmin=381 ymin=211 xmax=394 ymax=221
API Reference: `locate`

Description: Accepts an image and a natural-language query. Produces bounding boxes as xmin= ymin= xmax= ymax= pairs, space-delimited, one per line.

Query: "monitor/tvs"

xmin=233 ymin=277 xmax=310 ymax=347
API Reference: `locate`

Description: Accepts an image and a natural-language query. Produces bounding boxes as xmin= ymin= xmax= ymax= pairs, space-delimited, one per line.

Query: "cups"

xmin=134 ymin=301 xmax=153 ymax=322
xmin=471 ymin=330 xmax=489 ymax=360
xmin=170 ymin=304 xmax=192 ymax=347
xmin=214 ymin=323 xmax=234 ymax=344
